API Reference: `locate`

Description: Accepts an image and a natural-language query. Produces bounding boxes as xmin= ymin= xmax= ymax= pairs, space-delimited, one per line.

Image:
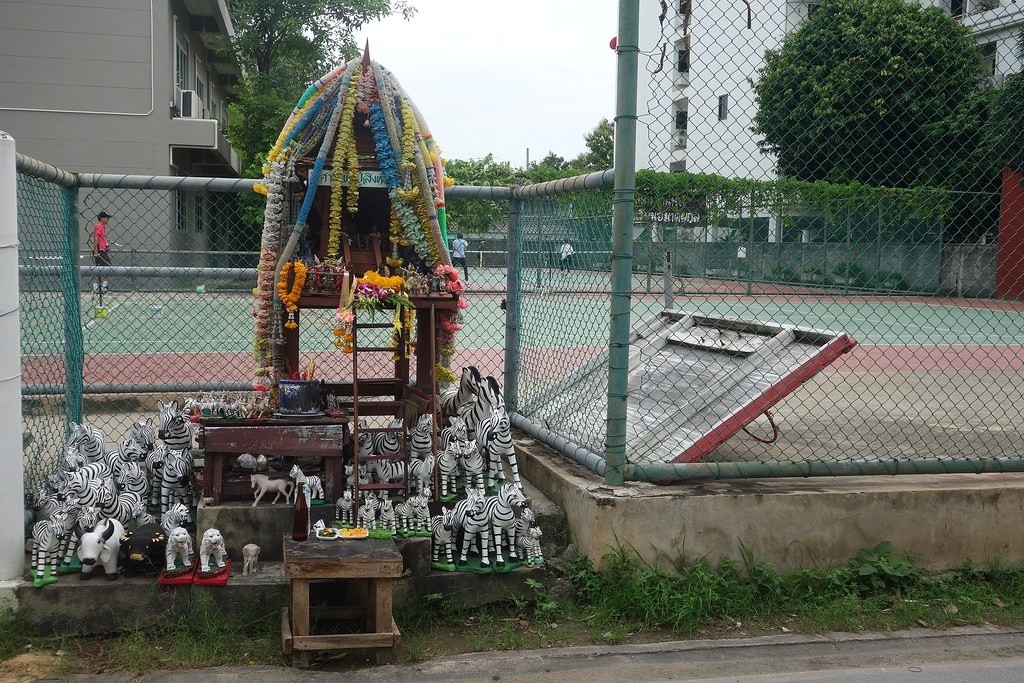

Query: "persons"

xmin=91 ymin=212 xmax=112 ymax=294
xmin=452 ymin=234 xmax=470 ymax=285
xmin=473 ymin=241 xmax=486 ymax=269
xmin=561 ymin=239 xmax=573 ymax=280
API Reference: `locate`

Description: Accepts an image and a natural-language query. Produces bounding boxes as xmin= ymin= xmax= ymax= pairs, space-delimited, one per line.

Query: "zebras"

xmin=31 ymin=366 xmax=543 ymax=581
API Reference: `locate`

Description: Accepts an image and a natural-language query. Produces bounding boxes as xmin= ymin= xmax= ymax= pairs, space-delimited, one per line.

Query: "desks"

xmin=279 ymin=532 xmax=404 ymax=665
xmin=190 ymin=404 xmax=350 ymax=507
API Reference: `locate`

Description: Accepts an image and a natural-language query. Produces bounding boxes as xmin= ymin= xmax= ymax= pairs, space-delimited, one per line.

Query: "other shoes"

xmin=464 ymin=281 xmax=471 ymax=285
xmin=101 ymin=286 xmax=112 ymax=294
xmin=92 ymin=285 xmax=100 ymax=293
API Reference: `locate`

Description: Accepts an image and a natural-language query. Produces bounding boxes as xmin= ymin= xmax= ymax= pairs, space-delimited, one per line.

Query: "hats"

xmin=98 ymin=212 xmax=113 ymax=220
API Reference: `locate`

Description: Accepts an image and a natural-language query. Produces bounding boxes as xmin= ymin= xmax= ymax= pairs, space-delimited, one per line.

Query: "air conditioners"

xmin=180 ymin=89 xmax=210 ymax=120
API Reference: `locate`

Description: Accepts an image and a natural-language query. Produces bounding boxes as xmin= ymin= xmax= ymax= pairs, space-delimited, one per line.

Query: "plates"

xmin=316 ymin=528 xmax=339 ymax=540
xmin=337 ymin=528 xmax=369 ymax=539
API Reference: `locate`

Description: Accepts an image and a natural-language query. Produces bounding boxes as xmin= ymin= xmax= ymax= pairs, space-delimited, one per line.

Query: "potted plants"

xmin=276 ymin=358 xmax=320 ymax=413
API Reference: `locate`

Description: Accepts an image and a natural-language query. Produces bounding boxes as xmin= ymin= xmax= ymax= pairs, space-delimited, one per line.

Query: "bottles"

xmin=293 ymin=482 xmax=308 ymax=541
xmin=294 ymin=479 xmax=311 ymax=535
